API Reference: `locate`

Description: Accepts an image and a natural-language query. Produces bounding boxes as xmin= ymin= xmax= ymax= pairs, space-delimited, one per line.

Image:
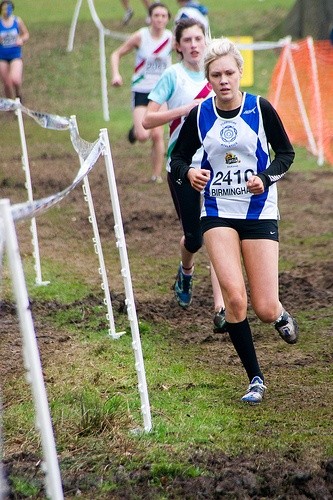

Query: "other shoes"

xmin=129 ymin=126 xmax=136 ymax=144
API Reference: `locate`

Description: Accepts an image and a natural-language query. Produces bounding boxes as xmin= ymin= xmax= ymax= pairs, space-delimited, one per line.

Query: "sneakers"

xmin=214 ymin=307 xmax=227 ymax=332
xmin=274 ymin=308 xmax=298 ymax=344
xmin=241 ymin=376 xmax=268 ymax=405
xmin=175 ymin=262 xmax=194 ymax=307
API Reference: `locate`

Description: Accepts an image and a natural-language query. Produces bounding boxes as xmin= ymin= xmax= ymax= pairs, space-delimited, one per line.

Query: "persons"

xmin=142 ymin=24 xmax=226 ymax=334
xmin=169 ymin=38 xmax=296 ymax=405
xmin=0 ymin=0 xmax=30 ymax=102
xmin=110 ymin=3 xmax=178 ymax=184
xmin=119 ymin=0 xmax=154 ymax=24
xmin=174 ymin=0 xmax=210 ymax=34
